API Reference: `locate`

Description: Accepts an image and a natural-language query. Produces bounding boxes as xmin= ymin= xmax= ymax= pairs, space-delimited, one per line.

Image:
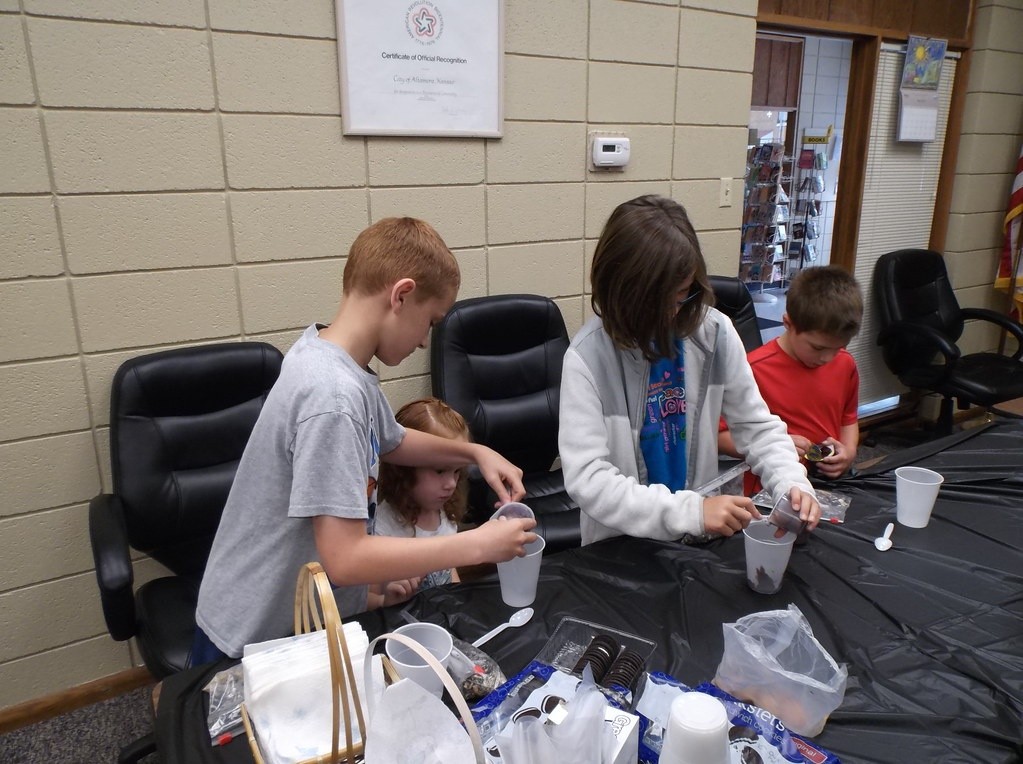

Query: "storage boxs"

xmin=532 ymin=615 xmax=657 ymax=691
xmin=604 ymin=705 xmax=640 ymax=764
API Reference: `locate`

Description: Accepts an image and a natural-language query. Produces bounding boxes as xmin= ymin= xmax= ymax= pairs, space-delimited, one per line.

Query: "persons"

xmin=186 ymin=217 xmax=537 ymax=669
xmin=558 ymin=197 xmax=822 ymax=542
xmin=366 ymin=398 xmax=471 ymax=608
xmin=717 ymin=262 xmax=865 ymax=495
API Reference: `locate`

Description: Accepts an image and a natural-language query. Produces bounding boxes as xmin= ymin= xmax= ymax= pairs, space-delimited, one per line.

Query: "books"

xmin=896 ymin=34 xmax=949 ymax=144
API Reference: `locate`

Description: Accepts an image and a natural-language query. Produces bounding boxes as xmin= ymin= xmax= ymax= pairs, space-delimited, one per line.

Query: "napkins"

xmin=241 ymin=621 xmax=382 ymax=764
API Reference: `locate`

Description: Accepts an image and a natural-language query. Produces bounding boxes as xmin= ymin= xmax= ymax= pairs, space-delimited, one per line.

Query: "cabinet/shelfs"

xmin=739 ymin=141 xmax=827 ymax=304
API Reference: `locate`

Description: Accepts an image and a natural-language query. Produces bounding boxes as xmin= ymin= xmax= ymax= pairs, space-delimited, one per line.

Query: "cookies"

xmin=729 ymin=725 xmax=764 ymax=764
xmin=572 ymin=634 xmax=646 ymax=694
xmin=487 ymin=695 xmax=568 ymax=758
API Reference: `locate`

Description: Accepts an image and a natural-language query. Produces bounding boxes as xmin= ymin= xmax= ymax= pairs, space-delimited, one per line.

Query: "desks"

xmin=146 ymin=418 xmax=1023 ymax=764
xmin=991 ymin=397 xmax=1023 ymax=419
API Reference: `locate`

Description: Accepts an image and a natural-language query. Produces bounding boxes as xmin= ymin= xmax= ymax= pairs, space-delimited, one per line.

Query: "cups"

xmin=489 ymin=502 xmax=535 ymax=531
xmin=894 ymin=466 xmax=945 ymax=528
xmin=788 ymin=149 xmax=830 ymax=262
xmin=385 ymin=621 xmax=454 ymax=703
xmin=496 ymin=534 xmax=546 ymax=608
xmin=657 ymin=690 xmax=739 ymax=764
xmin=742 ymin=521 xmax=798 ymax=595
xmin=766 ymin=490 xmax=806 ymax=535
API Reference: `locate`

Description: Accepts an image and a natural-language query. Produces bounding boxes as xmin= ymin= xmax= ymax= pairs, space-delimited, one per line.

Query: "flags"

xmin=993 ymin=143 xmax=1023 ymax=339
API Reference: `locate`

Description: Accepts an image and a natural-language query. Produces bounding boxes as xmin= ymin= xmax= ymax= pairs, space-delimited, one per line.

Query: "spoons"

xmin=470 ymin=606 xmax=535 ymax=648
xmin=873 ymin=522 xmax=895 ymax=552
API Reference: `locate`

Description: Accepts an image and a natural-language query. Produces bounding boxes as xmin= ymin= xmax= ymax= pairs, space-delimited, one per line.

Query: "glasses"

xmin=674 ymin=290 xmax=704 ymax=306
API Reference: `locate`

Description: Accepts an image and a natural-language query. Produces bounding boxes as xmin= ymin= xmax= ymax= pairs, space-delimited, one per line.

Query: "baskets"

xmin=236 ymin=560 xmax=401 ymax=764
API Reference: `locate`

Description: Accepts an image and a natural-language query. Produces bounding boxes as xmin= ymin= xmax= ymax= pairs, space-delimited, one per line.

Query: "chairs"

xmin=429 ymin=293 xmax=580 ymax=574
xmin=706 ymin=275 xmax=763 ymax=353
xmin=863 ymin=249 xmax=1023 ymax=448
xmin=90 ymin=342 xmax=285 ymax=681
